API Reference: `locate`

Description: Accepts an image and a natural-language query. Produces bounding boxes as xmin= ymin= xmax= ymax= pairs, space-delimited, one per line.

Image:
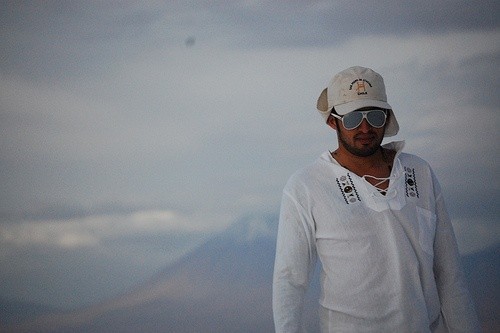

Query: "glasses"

xmin=330 ymin=110 xmax=389 ymax=131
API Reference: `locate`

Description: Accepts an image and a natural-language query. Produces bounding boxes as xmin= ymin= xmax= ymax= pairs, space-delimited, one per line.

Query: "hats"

xmin=317 ymin=66 xmax=400 ymax=137
